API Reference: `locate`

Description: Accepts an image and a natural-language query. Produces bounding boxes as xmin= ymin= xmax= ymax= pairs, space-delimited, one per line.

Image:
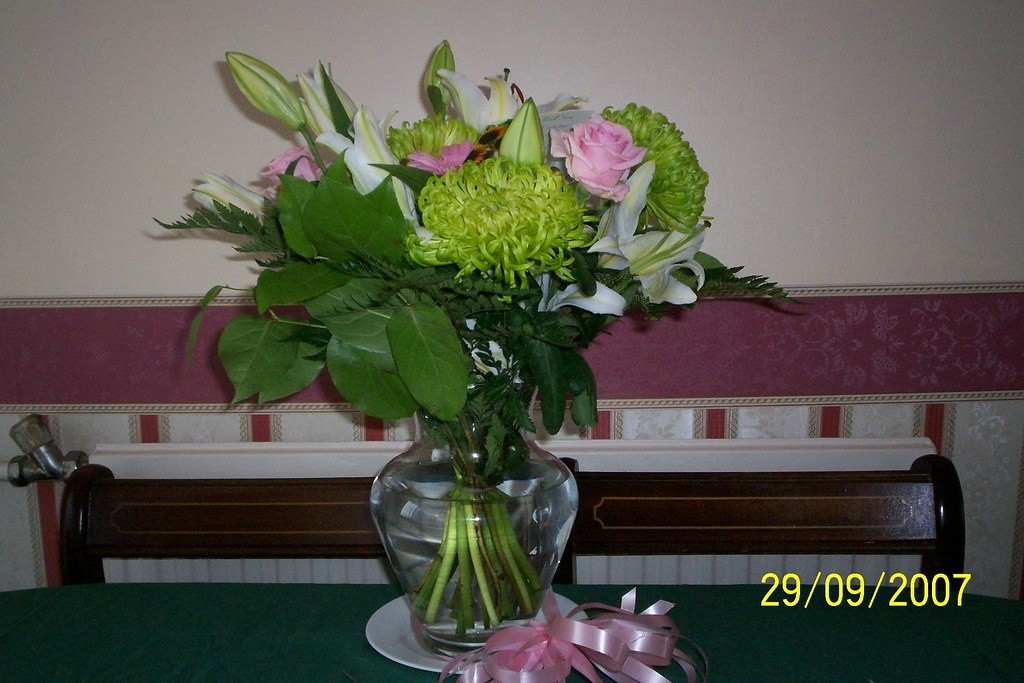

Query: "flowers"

xmin=154 ymin=38 xmax=812 ymax=638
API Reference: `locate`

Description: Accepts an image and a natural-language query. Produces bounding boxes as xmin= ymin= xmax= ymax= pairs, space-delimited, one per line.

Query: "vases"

xmin=366 ymin=400 xmax=580 ymax=653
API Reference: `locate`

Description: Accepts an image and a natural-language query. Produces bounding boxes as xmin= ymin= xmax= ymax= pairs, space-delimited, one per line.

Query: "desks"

xmin=0 ymin=585 xmax=1024 ymax=683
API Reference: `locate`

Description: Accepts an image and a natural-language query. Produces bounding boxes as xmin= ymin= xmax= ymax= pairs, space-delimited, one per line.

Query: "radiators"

xmin=0 ymin=415 xmax=936 ymax=586
